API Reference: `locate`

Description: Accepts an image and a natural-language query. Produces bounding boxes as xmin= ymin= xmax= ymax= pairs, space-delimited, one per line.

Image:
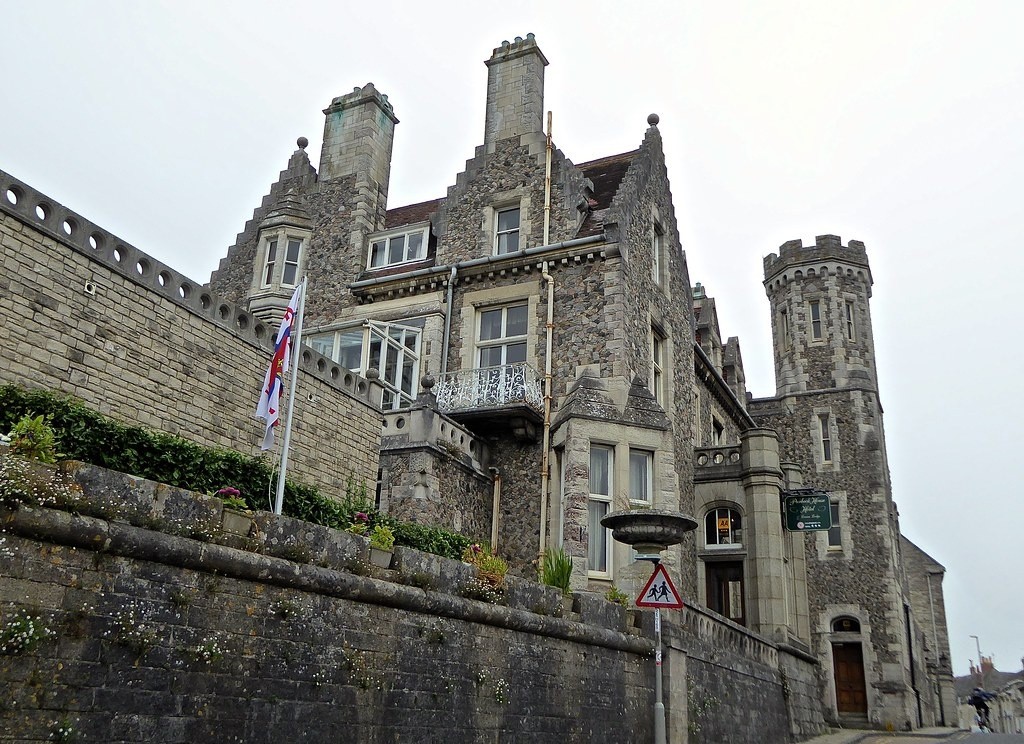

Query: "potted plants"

xmin=598 ymin=483 xmax=699 ymax=554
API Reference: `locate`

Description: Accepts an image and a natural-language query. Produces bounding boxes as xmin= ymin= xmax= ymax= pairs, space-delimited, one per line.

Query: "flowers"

xmin=606 ymin=585 xmax=635 ymax=610
xmin=532 ymin=547 xmax=576 ymax=599
xmin=11 ymin=411 xmax=67 ymax=464
xmin=460 ymin=541 xmax=508 ymax=576
xmin=346 ymin=513 xmax=396 ymax=550
xmin=208 ymin=485 xmax=256 ymax=514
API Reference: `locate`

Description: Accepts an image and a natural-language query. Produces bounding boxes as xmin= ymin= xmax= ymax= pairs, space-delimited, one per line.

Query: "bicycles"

xmin=974 ymin=699 xmax=996 ymax=734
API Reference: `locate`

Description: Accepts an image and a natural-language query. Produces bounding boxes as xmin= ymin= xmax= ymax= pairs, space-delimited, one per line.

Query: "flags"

xmin=256 ymin=284 xmax=302 ymax=451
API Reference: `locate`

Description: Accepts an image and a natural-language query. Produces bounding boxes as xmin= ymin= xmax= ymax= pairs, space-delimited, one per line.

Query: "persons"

xmin=972 ymin=682 xmax=992 ymax=729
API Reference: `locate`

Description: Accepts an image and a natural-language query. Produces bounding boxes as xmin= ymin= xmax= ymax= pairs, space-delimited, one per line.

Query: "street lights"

xmin=969 ymin=635 xmax=981 ymax=660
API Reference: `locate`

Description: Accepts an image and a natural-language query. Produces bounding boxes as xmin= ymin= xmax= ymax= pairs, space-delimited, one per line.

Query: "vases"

xmin=556 ymin=595 xmax=574 ymax=611
xmin=368 ymin=548 xmax=394 ymax=569
xmin=622 ymin=612 xmax=636 ymax=625
xmin=483 ymin=572 xmax=505 ymax=586
xmin=12 ymin=455 xmax=61 ymax=486
xmin=221 ymin=510 xmax=256 ymax=535
